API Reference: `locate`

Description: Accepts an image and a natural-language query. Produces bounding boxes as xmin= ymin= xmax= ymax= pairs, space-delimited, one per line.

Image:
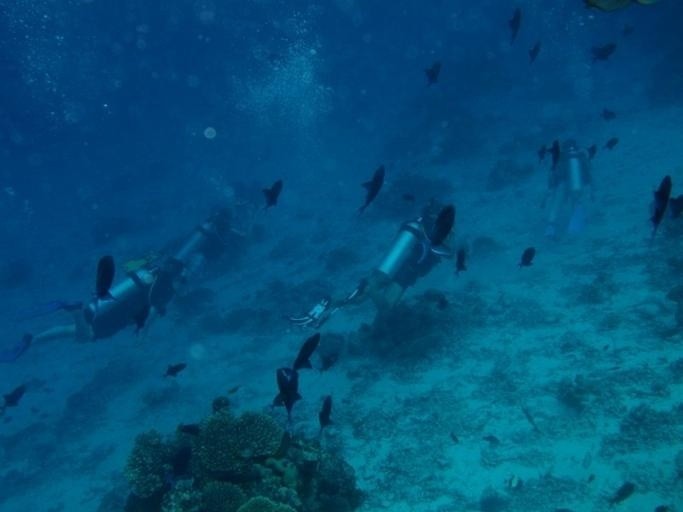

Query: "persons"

xmin=545 ymin=171 xmax=587 ymax=242
xmin=0 ymin=179 xmax=246 ymax=364
xmin=290 ymin=194 xmax=452 ymax=334
